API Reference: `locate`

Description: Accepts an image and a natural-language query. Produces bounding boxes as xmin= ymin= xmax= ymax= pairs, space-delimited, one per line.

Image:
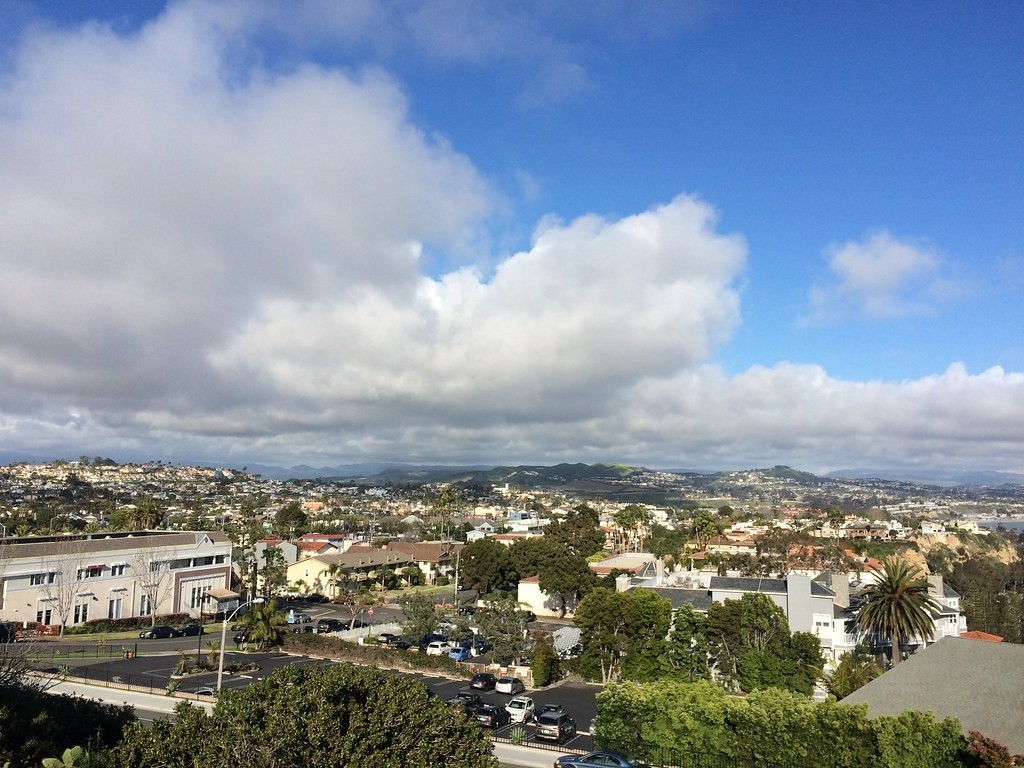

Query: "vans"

xmin=533 ymin=711 xmax=576 ymax=745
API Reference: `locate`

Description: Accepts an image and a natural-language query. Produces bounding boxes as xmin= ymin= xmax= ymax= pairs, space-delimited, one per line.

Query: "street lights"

xmin=215 ymin=597 xmax=265 ymax=698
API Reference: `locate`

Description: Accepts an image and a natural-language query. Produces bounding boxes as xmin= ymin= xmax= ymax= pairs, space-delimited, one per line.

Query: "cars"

xmin=468 ymin=672 xmax=499 ymax=692
xmin=445 ymin=691 xmax=511 ymax=730
xmin=231 ymin=587 xmax=538 ymax=662
xmin=504 ymin=696 xmax=536 ymax=724
xmin=554 ymin=749 xmax=653 ymax=768
xmin=139 ymin=625 xmax=178 ymax=640
xmin=175 ymin=623 xmax=205 ymax=637
xmin=494 ymin=676 xmax=525 ymax=695
xmin=532 ymin=704 xmax=564 ymax=724
